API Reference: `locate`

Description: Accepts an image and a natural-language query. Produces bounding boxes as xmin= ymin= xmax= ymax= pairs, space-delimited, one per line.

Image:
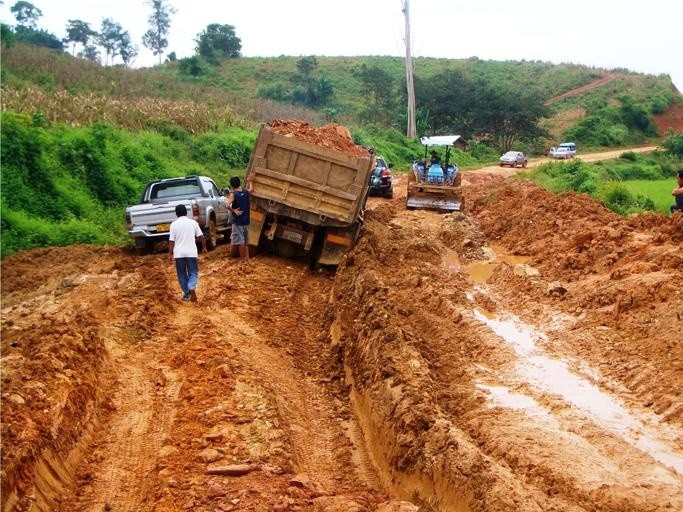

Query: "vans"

xmin=558 ymin=143 xmax=576 ymax=156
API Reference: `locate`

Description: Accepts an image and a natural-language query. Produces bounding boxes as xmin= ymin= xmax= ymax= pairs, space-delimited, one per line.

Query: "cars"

xmin=498 ymin=151 xmax=529 ymax=168
xmin=367 ymin=155 xmax=395 ymax=198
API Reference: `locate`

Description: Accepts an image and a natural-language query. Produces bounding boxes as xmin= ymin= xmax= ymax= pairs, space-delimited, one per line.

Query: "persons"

xmin=426 ymin=151 xmax=443 ymax=167
xmin=670 ymin=170 xmax=683 ymax=218
xmin=225 ymin=172 xmax=256 ymax=263
xmin=167 ymin=204 xmax=208 ymax=302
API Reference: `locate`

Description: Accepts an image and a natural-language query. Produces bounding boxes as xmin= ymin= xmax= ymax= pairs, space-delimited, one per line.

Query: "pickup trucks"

xmin=549 ymin=147 xmax=576 ymax=160
xmin=125 ymin=175 xmax=232 ymax=254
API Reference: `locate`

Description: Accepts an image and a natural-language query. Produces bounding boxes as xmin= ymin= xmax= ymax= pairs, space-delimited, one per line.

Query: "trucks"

xmin=230 ymin=119 xmax=377 ymax=275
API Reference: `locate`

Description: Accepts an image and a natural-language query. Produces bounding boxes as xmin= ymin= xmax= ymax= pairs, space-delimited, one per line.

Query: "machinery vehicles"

xmin=407 ymin=134 xmax=469 ymax=212
